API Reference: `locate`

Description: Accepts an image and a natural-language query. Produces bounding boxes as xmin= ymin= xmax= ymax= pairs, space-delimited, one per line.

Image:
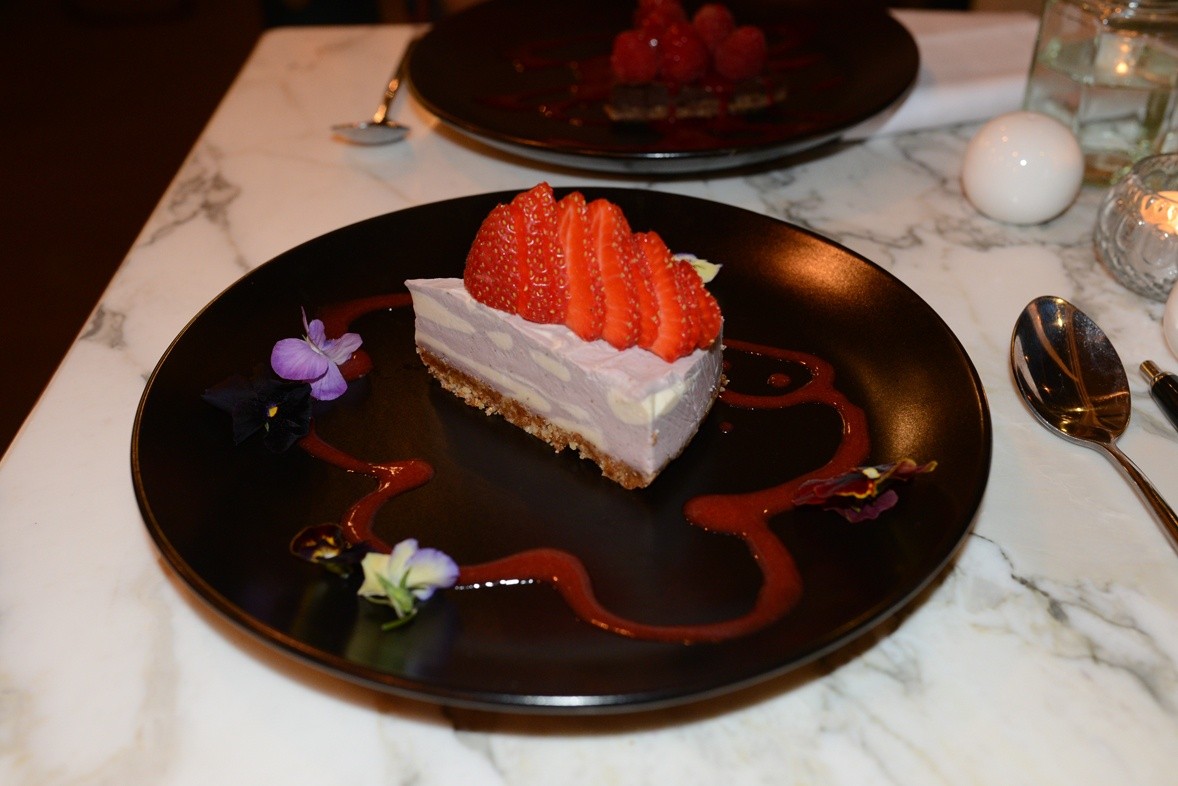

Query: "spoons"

xmin=1010 ymin=292 xmax=1177 ymax=562
xmin=332 ymin=33 xmax=415 ymax=149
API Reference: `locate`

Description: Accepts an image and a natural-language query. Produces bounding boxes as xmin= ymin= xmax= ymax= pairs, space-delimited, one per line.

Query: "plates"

xmin=128 ymin=186 xmax=995 ymax=721
xmin=403 ymin=2 xmax=924 ymax=180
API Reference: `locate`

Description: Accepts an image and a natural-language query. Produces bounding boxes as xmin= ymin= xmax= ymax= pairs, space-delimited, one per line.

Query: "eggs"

xmin=961 ymin=110 xmax=1084 ymax=225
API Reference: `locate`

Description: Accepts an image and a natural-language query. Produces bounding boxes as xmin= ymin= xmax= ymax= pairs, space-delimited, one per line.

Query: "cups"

xmin=1017 ymin=2 xmax=1176 ymax=197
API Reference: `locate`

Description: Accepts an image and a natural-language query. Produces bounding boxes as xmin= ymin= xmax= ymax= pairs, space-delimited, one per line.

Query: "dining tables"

xmin=3 ymin=10 xmax=1176 ymax=786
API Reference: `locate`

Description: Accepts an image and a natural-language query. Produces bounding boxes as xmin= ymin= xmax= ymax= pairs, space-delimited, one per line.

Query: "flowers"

xmin=268 ymin=304 xmax=364 ymax=404
xmin=357 ymin=537 xmax=462 ymax=633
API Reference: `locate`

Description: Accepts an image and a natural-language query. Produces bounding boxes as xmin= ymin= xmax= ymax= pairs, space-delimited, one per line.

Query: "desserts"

xmin=403 ymin=183 xmax=725 ymax=492
xmin=604 ymin=1 xmax=792 ymax=120
